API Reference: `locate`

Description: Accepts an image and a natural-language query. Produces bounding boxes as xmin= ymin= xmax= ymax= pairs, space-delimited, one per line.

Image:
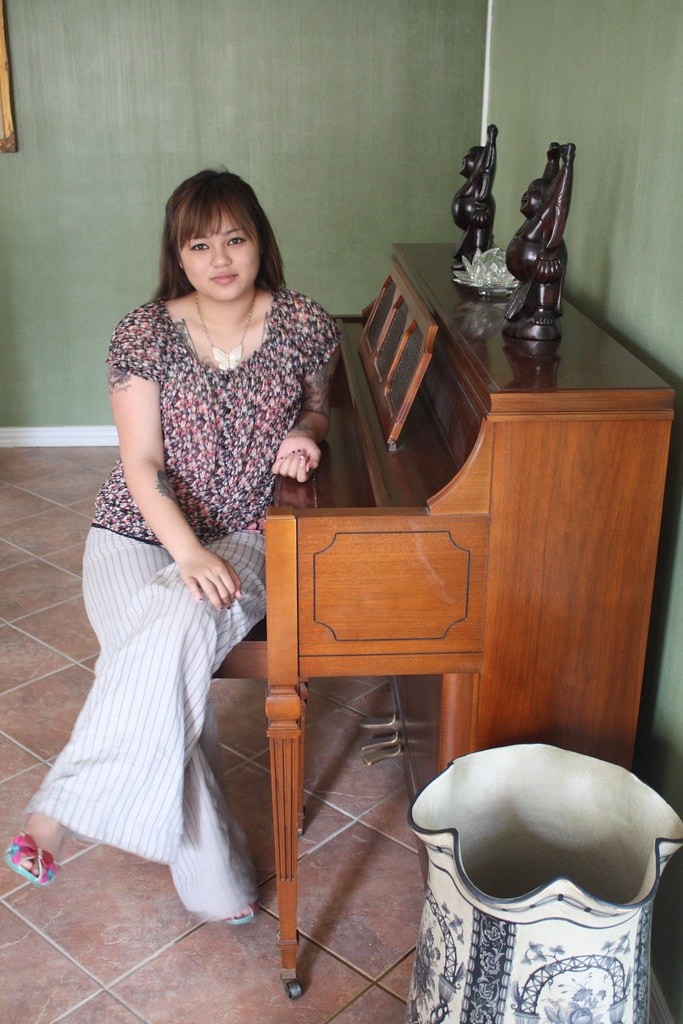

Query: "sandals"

xmin=3 ymin=828 xmax=63 ymax=887
xmin=222 ymin=898 xmax=261 ymax=925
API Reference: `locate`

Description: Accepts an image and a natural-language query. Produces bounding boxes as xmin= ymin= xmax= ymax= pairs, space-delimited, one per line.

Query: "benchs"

xmin=214 ymin=614 xmax=308 ymax=835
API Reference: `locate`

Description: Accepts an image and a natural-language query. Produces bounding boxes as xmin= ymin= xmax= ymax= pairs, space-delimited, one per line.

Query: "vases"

xmin=409 ymin=741 xmax=683 ymax=1024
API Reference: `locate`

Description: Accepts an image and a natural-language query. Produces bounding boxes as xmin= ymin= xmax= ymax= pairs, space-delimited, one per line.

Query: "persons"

xmin=3 ymin=171 xmax=342 ymax=923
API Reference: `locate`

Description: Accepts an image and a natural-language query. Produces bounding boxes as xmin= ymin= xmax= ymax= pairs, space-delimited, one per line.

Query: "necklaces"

xmin=194 ymin=291 xmax=257 ymax=372
